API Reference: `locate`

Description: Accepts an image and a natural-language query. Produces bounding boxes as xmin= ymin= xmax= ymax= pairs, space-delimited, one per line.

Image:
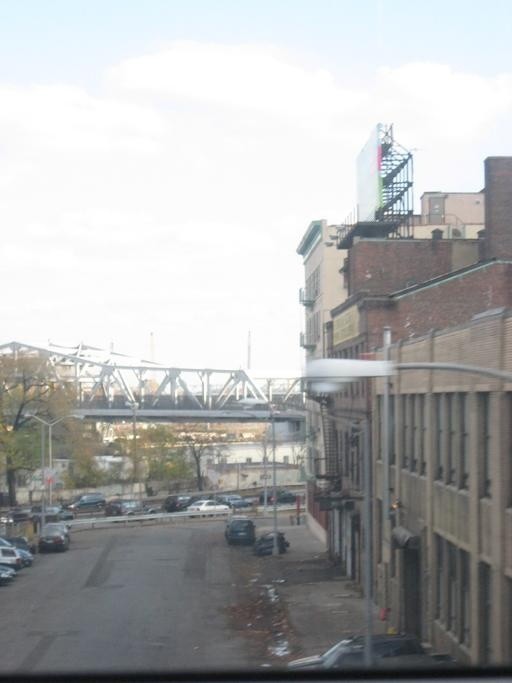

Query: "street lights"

xmin=24 ymin=411 xmax=85 ymax=505
xmin=300 ymin=355 xmax=511 ymax=392
xmin=238 ymin=395 xmax=376 ymax=669
xmin=217 ymin=409 xmax=270 ymax=519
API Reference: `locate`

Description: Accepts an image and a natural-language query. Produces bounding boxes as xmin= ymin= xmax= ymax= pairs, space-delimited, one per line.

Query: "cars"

xmin=64 ymin=486 xmax=296 ymax=521
xmin=0 ymin=498 xmax=76 ymax=583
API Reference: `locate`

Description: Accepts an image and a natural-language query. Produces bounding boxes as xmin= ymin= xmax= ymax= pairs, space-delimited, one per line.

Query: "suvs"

xmin=286 ymin=630 xmax=459 ymax=669
xmin=223 ymin=517 xmax=256 ymax=546
